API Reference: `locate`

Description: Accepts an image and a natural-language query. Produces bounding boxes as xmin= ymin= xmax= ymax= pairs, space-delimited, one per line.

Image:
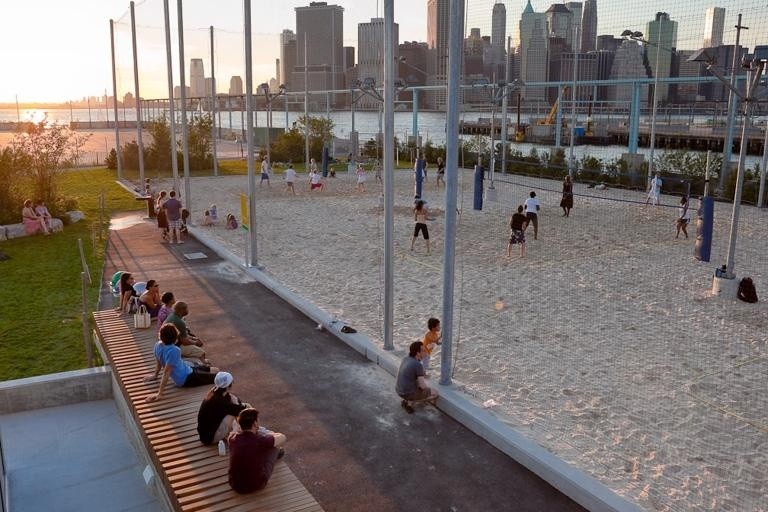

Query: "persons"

xmin=348 ymin=152 xmax=383 ymax=192
xmin=35 ymin=198 xmax=55 ymax=233
xmin=411 ymin=200 xmax=430 ymax=254
xmin=22 ymin=199 xmax=50 ymax=236
xmin=204 ymin=204 xmax=238 ymax=229
xmin=143 ymin=323 xmax=220 ymax=402
xmin=227 ymin=408 xmax=288 ymax=495
xmin=395 ymin=342 xmax=438 ymax=408
xmin=115 ymin=273 xmax=205 ymax=360
xmin=645 ymin=172 xmax=662 ymax=210
xmin=197 ymin=371 xmax=252 ymax=445
xmin=420 ymin=318 xmax=442 ymax=376
xmin=423 ymin=153 xmax=428 ymax=181
xmin=676 ymin=197 xmax=690 ymax=238
xmin=260 ymin=155 xmax=323 ymax=194
xmin=436 ymin=157 xmax=446 ymax=187
xmin=157 ymin=190 xmax=189 ymax=244
xmin=507 ymin=175 xmax=573 ymax=257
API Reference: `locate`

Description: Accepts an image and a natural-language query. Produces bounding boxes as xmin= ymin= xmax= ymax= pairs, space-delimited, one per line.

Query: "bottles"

xmin=714 ymin=267 xmax=726 ymax=277
xmin=217 ymin=440 xmax=225 ymax=457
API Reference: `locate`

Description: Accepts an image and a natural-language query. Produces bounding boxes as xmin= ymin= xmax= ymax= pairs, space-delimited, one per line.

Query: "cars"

xmin=735 ymin=115 xmax=763 ymax=124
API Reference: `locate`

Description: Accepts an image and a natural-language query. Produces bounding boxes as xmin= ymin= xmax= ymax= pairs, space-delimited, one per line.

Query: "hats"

xmin=214 ymin=371 xmax=233 ymax=388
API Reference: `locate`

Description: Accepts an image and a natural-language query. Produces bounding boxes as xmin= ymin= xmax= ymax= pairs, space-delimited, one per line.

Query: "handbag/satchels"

xmin=737 ymin=278 xmax=757 ymax=303
xmin=134 ymin=305 xmax=151 ymax=329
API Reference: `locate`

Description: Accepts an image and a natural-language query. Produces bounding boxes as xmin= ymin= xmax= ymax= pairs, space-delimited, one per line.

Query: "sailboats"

xmin=194 ymin=101 xmax=204 ymax=114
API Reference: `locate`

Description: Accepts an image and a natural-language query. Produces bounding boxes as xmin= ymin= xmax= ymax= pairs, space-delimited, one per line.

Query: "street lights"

xmin=686 ymin=46 xmax=766 ymax=300
xmin=258 ymin=79 xmax=288 ymax=167
xmin=620 ymin=9 xmax=681 ymax=199
xmin=350 ymin=52 xmax=409 ymax=134
xmin=469 ymin=76 xmax=525 ymax=202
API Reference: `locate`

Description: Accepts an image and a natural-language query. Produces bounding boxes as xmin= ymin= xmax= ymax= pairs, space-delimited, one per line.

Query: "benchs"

xmin=91 ymin=306 xmax=327 ymax=512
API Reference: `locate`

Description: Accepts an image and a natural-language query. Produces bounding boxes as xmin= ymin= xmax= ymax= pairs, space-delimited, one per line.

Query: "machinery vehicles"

xmin=536 ymin=86 xmax=569 ymax=127
